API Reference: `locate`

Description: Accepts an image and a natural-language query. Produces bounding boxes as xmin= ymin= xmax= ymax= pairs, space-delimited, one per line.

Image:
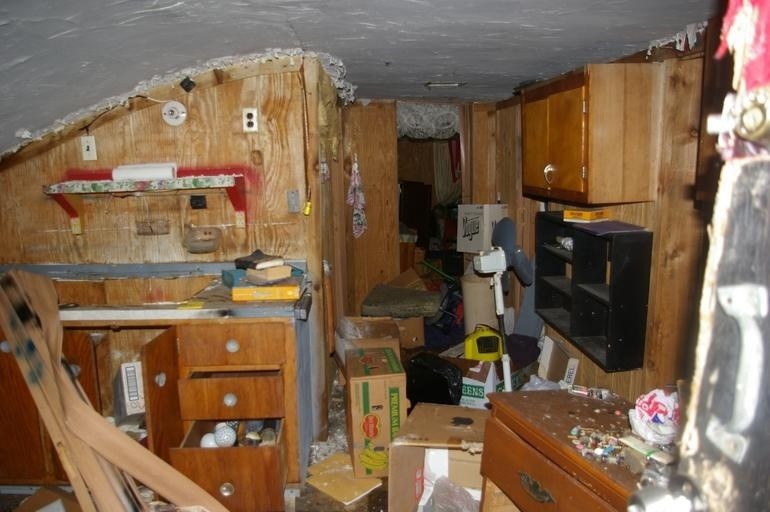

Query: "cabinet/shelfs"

xmin=521 ymin=63 xmax=663 ymax=208
xmin=0 ymin=318 xmax=297 ymax=512
xmin=534 ymin=211 xmax=652 ymax=373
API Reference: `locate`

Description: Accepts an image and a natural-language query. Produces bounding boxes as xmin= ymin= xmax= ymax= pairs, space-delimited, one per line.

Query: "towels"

xmin=348 ymin=162 xmax=367 ymax=238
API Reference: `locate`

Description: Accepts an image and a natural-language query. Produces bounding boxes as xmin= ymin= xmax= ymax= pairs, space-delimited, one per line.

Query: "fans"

xmin=473 ymin=217 xmax=532 ymax=392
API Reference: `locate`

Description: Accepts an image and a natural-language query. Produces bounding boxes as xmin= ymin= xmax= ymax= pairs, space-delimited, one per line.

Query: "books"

xmin=237 ymin=248 xmax=286 ymax=270
xmin=244 ymin=264 xmax=292 ymax=282
xmin=306 ymin=448 xmax=353 ymax=477
xmin=305 ymin=461 xmax=385 ymax=503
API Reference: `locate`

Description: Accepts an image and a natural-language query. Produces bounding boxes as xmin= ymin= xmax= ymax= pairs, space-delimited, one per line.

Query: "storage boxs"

xmin=456 ymin=203 xmax=509 ymax=254
xmin=113 ymin=362 xmax=145 ymax=426
xmin=334 ymin=270 xmax=485 ymax=512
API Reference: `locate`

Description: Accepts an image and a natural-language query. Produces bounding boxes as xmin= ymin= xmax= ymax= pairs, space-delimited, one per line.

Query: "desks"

xmin=479 ymin=392 xmax=646 ymax=512
